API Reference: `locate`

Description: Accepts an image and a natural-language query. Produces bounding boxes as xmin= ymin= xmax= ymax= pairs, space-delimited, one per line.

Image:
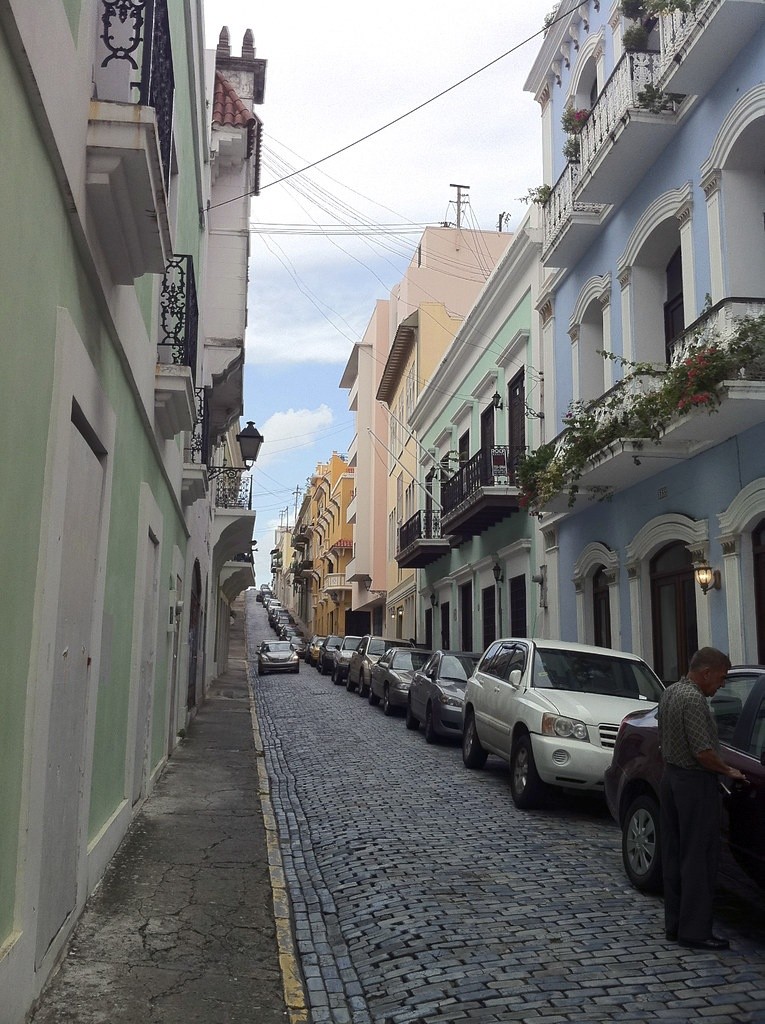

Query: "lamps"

xmin=491 ymin=563 xmax=504 ymax=584
xmin=391 ymin=614 xmax=395 ymax=619
xmin=398 ymin=609 xmax=404 ymax=615
xmin=429 ymin=592 xmax=438 ymax=607
xmin=236 ymin=421 xmax=263 ymax=468
xmin=691 ymin=552 xmax=722 ymax=596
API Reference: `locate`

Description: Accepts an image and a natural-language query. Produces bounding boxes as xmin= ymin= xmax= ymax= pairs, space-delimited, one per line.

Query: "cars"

xmin=601 ymin=664 xmax=765 ymax=914
xmin=331 ymin=636 xmax=362 ymax=684
xmin=288 ymin=636 xmax=311 ymax=659
xmin=255 ymin=640 xmax=300 ymax=676
xmin=310 ymin=637 xmax=326 ymax=668
xmin=316 ymin=635 xmax=344 ymax=674
xmin=249 ymin=584 xmax=305 ymax=642
xmin=369 ymin=647 xmax=437 ymax=715
xmin=407 ymin=650 xmax=487 ymax=743
xmin=305 ymin=634 xmax=322 ymax=664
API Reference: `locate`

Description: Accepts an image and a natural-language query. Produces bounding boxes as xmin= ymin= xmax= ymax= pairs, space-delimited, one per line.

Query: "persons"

xmin=657 ymin=646 xmax=747 ymax=950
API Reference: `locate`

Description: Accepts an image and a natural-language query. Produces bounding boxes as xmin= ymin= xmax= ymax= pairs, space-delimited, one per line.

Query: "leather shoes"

xmin=682 ymin=934 xmax=731 ymax=949
xmin=664 ymin=928 xmax=681 ymax=941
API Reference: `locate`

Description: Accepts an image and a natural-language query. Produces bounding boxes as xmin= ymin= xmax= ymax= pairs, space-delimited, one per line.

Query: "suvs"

xmin=462 ymin=638 xmax=669 ymax=808
xmin=347 ymin=633 xmax=417 ymax=697
